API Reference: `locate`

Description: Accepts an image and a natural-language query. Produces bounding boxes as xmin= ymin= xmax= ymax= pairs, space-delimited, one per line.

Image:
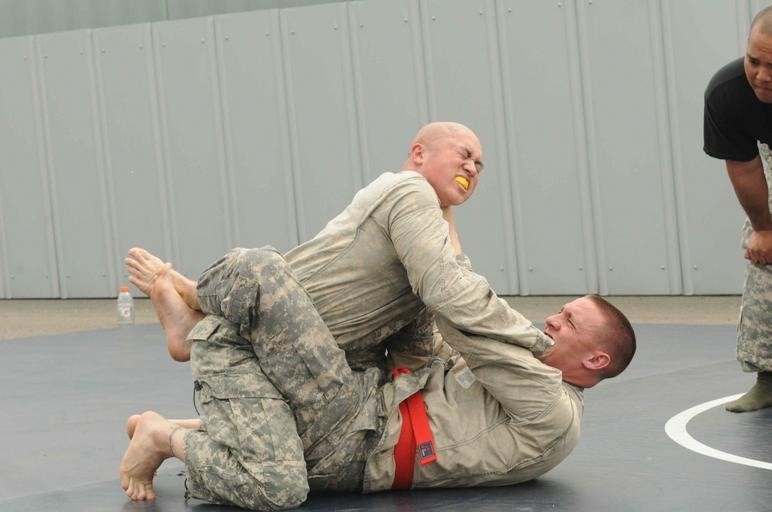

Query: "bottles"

xmin=116 ymin=286 xmax=135 ymax=325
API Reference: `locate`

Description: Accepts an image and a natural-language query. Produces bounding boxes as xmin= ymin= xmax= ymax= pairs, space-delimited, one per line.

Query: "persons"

xmin=126 ymin=246 xmax=636 ymax=493
xmin=121 ymin=121 xmax=553 ymax=512
xmin=703 ymin=6 xmax=772 ymax=411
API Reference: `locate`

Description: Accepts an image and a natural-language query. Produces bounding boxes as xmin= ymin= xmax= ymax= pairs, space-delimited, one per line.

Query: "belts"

xmin=391 ymin=367 xmax=438 ymax=490
